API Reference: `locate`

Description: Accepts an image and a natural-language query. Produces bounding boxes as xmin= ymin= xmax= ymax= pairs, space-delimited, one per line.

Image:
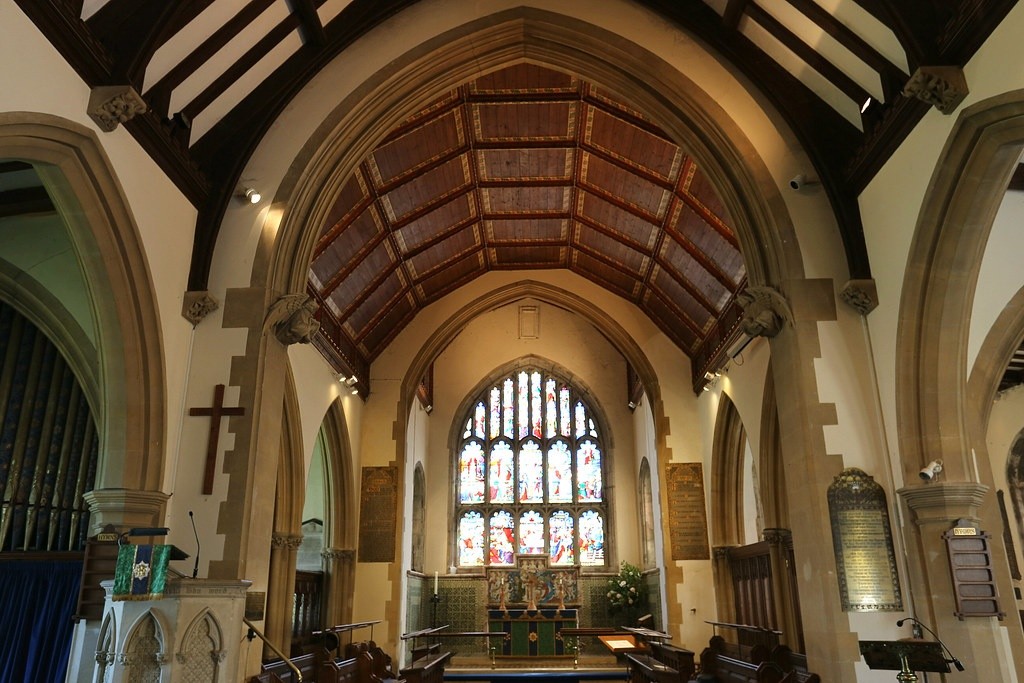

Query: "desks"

xmin=399 ymin=651 xmax=450 ymax=683
xmin=648 ymin=641 xmax=695 ymax=683
xmin=623 ymin=640 xmax=696 ymax=683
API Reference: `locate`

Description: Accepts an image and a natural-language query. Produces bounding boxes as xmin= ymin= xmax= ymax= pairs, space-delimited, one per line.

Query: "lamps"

xmin=335 ymin=372 xmax=347 ymax=383
xmin=704 ymin=383 xmax=711 ymax=392
xmin=704 ymin=371 xmax=715 ymax=382
xmin=346 ymin=374 xmax=358 ymax=386
xmin=245 ymin=187 xmax=261 ymax=204
xmin=350 ymin=386 xmax=358 ymax=395
xmin=715 ymin=368 xmax=725 ymax=376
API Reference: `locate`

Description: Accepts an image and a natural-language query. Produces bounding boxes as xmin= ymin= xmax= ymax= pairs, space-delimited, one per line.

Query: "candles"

xmin=434 ymin=570 xmax=439 ymax=598
xmin=559 ymin=578 xmax=562 ymax=585
xmin=501 ymin=577 xmax=504 ymax=585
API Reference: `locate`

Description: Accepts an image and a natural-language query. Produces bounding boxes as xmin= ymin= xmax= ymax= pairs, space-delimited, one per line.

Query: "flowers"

xmin=606 ymin=560 xmax=643 ymax=619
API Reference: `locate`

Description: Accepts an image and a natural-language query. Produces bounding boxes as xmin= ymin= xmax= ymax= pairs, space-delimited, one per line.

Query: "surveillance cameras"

xmin=790 ymin=175 xmax=805 ymax=190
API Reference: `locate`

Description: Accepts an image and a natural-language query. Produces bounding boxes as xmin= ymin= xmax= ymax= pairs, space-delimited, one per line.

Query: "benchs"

xmin=314 ymin=647 xmax=408 ymax=683
xmin=411 ymin=642 xmax=442 ymax=662
xmin=696 ymin=647 xmax=785 ymax=683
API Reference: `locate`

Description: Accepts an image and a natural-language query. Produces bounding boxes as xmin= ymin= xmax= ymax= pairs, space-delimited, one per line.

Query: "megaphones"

xmin=919 ymin=462 xmax=937 ymax=481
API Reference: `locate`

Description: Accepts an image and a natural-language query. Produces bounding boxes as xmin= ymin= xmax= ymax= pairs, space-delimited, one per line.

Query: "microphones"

xmin=188 ymin=510 xmax=200 ymax=577
xmin=897 ymin=617 xmax=965 ymax=672
